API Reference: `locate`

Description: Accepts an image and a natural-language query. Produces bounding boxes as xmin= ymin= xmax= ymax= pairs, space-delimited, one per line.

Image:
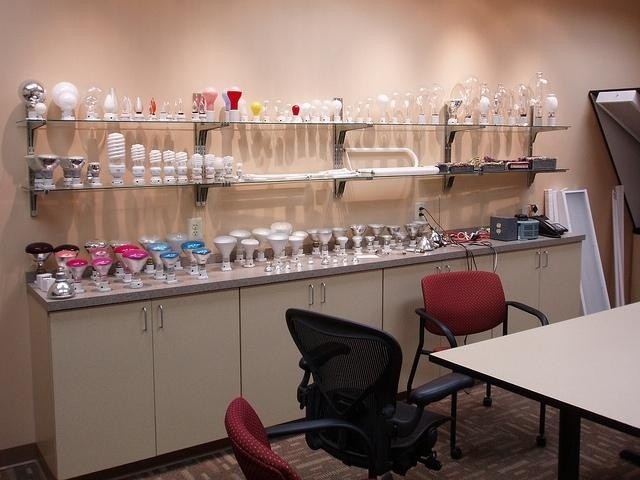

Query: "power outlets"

xmin=188 ymin=217 xmax=202 ymax=241
xmin=414 ymin=201 xmax=425 ymax=220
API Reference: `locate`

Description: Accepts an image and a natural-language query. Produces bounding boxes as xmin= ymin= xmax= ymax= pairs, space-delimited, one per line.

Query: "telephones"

xmin=530 ymin=215 xmax=568 ymax=236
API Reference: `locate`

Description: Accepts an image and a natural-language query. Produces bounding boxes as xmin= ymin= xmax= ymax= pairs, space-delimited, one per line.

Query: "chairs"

xmin=405 ymin=271 xmax=549 ymax=460
xmin=285 ymin=308 xmax=474 ymax=480
xmin=225 ymin=397 xmax=377 ymax=480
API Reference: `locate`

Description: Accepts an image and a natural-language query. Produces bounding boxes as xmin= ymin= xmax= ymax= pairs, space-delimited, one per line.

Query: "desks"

xmin=430 ymin=302 xmax=640 ymax=480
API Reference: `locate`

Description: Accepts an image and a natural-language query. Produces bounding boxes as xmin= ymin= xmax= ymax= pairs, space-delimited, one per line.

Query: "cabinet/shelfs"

xmin=27 ymin=288 xmax=239 ymax=480
xmin=239 ymin=267 xmax=383 ymax=428
xmin=17 ymin=118 xmax=570 ymax=217
xmin=383 ymin=255 xmax=492 ymax=399
xmin=492 ymin=242 xmax=582 ymax=338
